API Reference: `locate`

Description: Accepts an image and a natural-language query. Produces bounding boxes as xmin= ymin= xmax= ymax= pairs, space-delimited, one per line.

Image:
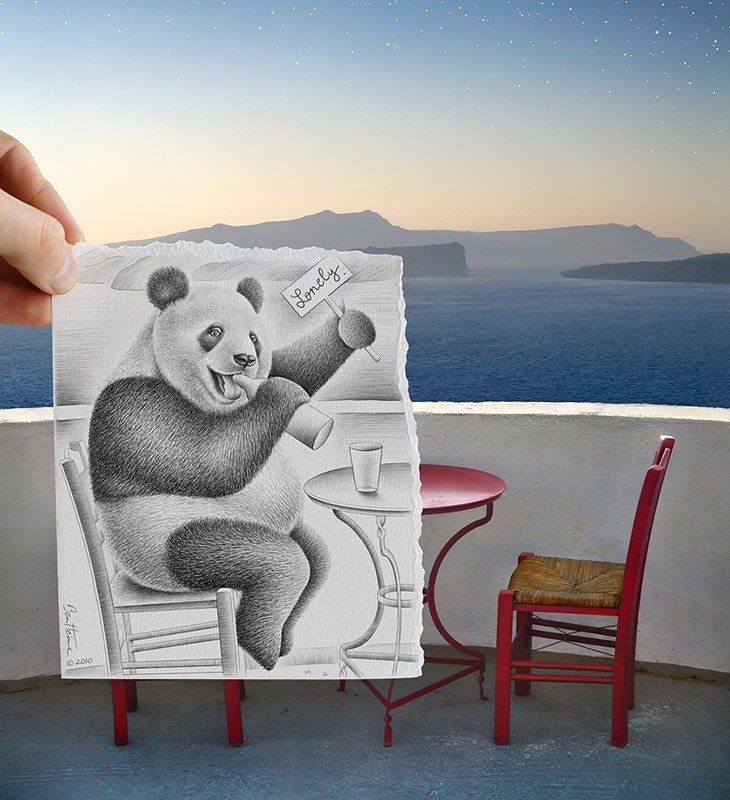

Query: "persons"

xmin=0 ymin=129 xmax=84 ymax=326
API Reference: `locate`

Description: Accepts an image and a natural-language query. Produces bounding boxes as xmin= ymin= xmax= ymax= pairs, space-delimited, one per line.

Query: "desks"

xmin=337 ymin=463 xmax=505 ymax=747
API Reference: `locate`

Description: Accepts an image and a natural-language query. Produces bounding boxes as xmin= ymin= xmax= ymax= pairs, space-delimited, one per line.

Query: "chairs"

xmin=493 ymin=436 xmax=675 ymax=745
